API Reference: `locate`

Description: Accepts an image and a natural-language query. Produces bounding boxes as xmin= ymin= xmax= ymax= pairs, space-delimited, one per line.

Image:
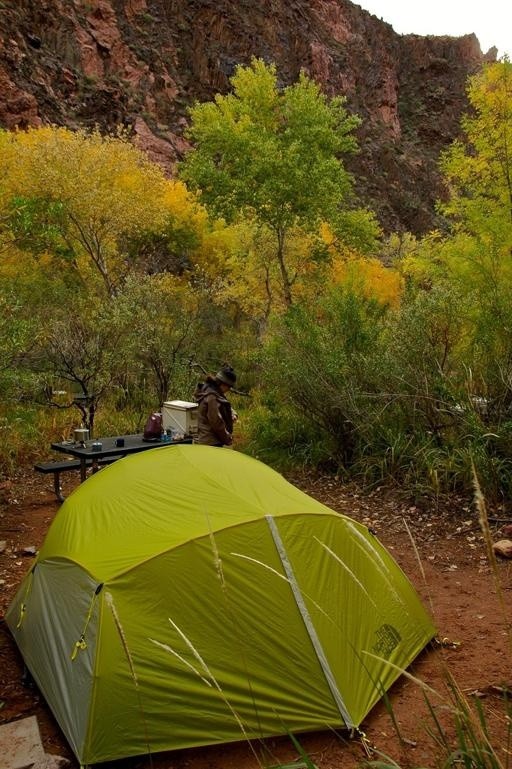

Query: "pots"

xmin=74 ymin=427 xmax=90 ymax=441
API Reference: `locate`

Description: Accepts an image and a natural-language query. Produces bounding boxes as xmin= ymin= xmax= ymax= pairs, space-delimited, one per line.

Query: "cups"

xmin=92 ymin=442 xmax=103 ymax=452
xmin=115 ymin=438 xmax=125 ymax=447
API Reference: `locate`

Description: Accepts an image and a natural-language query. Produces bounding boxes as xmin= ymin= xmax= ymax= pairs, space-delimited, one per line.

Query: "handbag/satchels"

xmin=143 ymin=413 xmax=164 ymax=438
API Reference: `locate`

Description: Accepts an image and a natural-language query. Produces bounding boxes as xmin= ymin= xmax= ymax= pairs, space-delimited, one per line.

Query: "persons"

xmin=191 ymin=369 xmax=239 ymax=448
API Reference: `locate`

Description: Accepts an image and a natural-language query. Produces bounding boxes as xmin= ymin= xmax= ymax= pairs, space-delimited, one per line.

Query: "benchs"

xmin=34 ymin=455 xmax=122 ymax=502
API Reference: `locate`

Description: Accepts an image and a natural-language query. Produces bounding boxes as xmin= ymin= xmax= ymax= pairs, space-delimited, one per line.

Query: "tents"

xmin=0 ymin=442 xmax=441 ymax=769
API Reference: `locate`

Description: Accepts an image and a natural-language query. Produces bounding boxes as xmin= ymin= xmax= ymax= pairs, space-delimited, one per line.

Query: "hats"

xmin=215 ymin=366 xmax=236 ymax=387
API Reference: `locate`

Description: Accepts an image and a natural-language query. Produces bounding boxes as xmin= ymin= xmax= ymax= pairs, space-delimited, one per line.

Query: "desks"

xmin=50 ymin=433 xmax=193 ymax=483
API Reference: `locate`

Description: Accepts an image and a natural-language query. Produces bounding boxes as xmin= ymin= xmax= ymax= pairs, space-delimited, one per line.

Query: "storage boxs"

xmin=162 ymin=399 xmax=200 ymax=438
